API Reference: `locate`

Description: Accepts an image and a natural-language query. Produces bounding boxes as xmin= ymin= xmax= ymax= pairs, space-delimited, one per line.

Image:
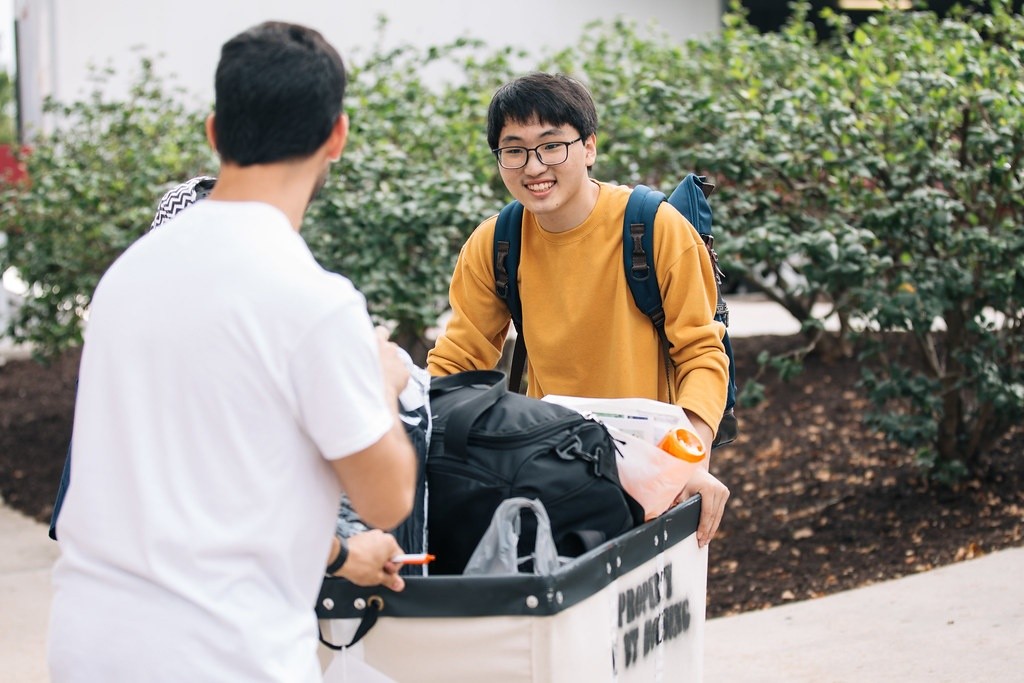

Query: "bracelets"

xmin=327 ymin=534 xmax=349 ymax=574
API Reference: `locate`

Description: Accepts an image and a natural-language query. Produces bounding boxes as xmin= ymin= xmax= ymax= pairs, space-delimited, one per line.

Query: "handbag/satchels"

xmin=541 ymin=392 xmax=708 ymax=519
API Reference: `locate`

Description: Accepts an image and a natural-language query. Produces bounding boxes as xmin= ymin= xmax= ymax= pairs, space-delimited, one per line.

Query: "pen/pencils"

xmin=393 ymin=552 xmax=436 ymax=565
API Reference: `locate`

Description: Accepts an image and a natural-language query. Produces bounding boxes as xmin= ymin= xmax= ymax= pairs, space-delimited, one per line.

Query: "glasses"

xmin=491 ymin=137 xmax=582 ymax=169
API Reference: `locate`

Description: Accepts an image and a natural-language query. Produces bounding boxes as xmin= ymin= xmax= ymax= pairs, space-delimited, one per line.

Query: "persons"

xmin=48 ymin=176 xmax=216 ymax=540
xmin=47 ymin=21 xmax=418 ymax=682
xmin=426 ymin=74 xmax=731 ymax=547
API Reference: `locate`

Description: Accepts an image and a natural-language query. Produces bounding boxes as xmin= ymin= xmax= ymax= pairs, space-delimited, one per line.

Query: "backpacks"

xmin=493 ymin=174 xmax=739 ymax=448
xmin=321 ymin=342 xmax=631 ymax=573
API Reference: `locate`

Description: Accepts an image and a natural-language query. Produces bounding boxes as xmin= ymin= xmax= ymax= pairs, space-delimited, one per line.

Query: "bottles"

xmin=657 ymin=428 xmax=706 ymax=463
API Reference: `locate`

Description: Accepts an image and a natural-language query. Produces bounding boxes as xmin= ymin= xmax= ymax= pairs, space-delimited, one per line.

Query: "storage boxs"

xmin=313 ymin=490 xmax=710 ymax=681
